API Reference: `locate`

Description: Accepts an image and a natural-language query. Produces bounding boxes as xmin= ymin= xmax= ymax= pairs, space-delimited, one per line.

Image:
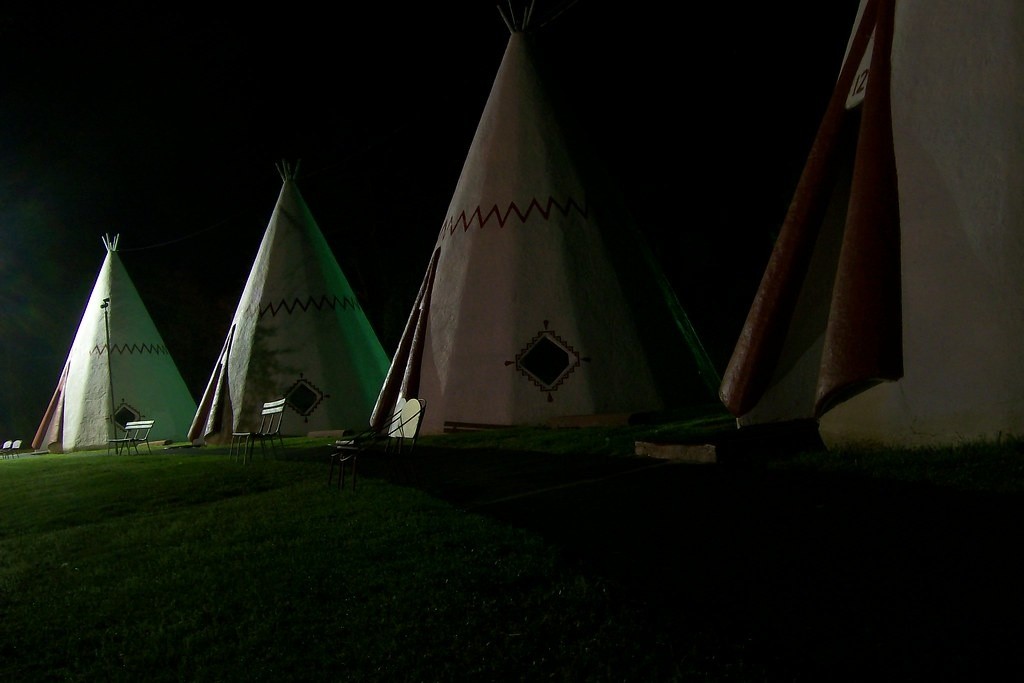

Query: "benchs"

xmin=108 ymin=420 xmax=155 ymax=457
xmin=229 ymin=398 xmax=287 ymax=465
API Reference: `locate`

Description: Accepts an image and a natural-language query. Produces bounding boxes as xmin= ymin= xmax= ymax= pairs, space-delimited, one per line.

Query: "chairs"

xmin=328 ymin=397 xmax=427 ymax=491
xmin=0 ymin=440 xmax=23 ymax=460
